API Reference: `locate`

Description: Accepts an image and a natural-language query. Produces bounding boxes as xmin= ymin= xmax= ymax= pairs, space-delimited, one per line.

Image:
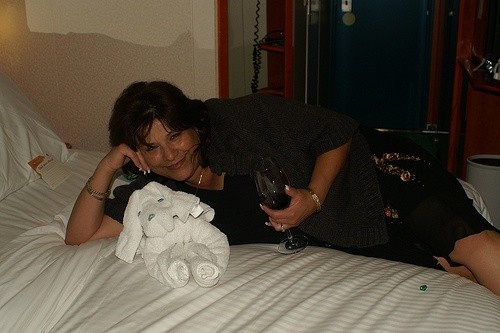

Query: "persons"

xmin=65 ymin=80 xmax=500 ymax=295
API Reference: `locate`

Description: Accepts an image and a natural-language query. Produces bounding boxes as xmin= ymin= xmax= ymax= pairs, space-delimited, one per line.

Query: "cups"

xmin=493 ymin=58 xmax=500 ymax=80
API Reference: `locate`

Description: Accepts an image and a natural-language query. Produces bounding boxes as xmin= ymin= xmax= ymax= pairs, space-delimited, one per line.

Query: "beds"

xmin=0 ymin=150 xmax=500 ymax=333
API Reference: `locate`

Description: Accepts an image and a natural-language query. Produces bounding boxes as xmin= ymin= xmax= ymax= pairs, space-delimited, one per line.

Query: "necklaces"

xmin=194 ymin=170 xmax=205 ymax=197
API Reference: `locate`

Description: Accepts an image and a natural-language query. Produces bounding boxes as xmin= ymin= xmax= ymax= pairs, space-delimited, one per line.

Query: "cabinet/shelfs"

xmin=254 ymin=0 xmax=296 ymax=102
xmin=460 ymin=79 xmax=500 ymax=183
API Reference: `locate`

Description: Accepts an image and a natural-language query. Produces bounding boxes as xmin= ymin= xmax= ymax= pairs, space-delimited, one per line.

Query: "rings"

xmin=281 ymin=223 xmax=285 ymax=234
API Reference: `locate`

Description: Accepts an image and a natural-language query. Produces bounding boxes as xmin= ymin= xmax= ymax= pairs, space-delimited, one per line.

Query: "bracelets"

xmin=85 ymin=176 xmax=111 ymax=200
xmin=305 ymin=187 xmax=322 ymax=214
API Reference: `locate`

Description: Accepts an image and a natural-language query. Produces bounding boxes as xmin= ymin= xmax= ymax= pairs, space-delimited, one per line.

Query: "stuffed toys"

xmin=117 ymin=182 xmax=229 ymax=288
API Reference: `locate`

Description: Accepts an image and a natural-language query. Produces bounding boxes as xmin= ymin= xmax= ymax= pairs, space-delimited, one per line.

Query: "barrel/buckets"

xmin=466 ymin=154 xmax=500 ymax=230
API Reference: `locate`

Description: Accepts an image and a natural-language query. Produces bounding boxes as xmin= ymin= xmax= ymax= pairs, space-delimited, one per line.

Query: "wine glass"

xmin=254 ymin=159 xmax=308 ymax=256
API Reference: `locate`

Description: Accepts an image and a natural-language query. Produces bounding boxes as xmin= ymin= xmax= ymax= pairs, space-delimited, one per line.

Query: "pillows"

xmin=0 ymin=69 xmax=80 ymax=202
